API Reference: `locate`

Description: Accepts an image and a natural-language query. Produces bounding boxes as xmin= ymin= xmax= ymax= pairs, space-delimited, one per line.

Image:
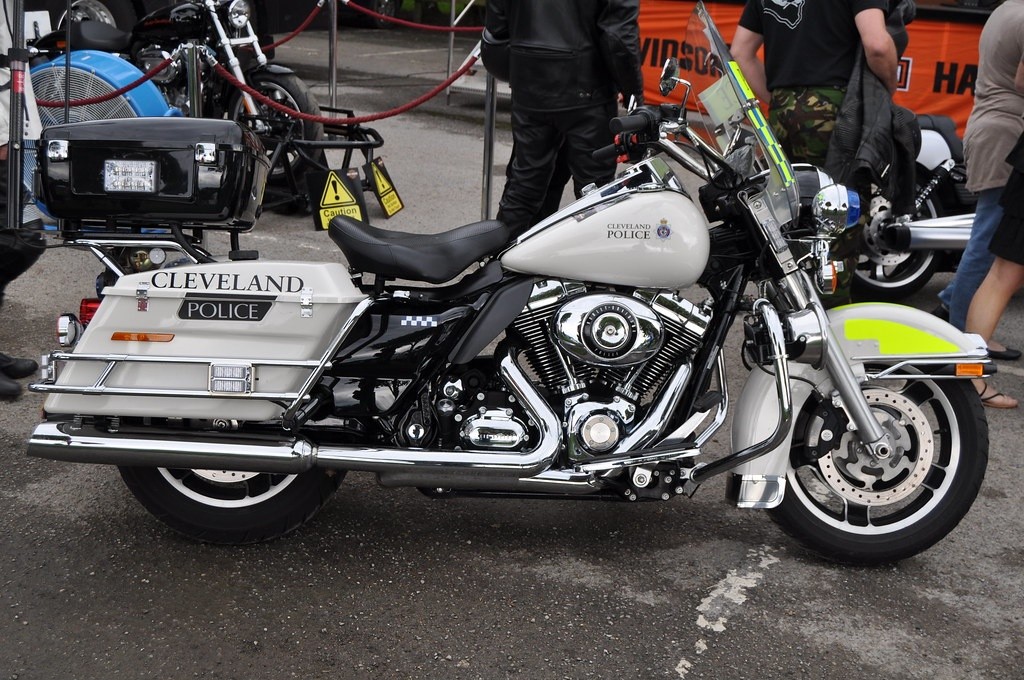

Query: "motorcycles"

xmin=24 ymin=3 xmax=996 ymax=568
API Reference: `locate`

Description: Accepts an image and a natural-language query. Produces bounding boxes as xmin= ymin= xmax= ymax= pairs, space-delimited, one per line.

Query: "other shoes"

xmin=984 ymin=342 xmax=1020 ymax=361
xmin=934 ymin=304 xmax=953 ymax=321
xmin=0 ymin=352 xmax=38 ymax=378
xmin=0 ymin=371 xmax=22 ymax=397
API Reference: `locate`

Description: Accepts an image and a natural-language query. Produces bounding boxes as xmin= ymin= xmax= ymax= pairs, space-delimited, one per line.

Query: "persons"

xmin=729 ymin=0 xmax=898 ymax=309
xmin=0 ymin=0 xmax=50 ymax=401
xmin=930 ymin=0 xmax=1024 ymax=333
xmin=481 ymin=0 xmax=644 ymax=225
xmin=964 ymin=132 xmax=1024 ymax=408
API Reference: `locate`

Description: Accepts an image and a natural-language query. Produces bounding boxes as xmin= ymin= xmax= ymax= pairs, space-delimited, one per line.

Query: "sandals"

xmin=977 ymin=382 xmax=1018 ymax=408
